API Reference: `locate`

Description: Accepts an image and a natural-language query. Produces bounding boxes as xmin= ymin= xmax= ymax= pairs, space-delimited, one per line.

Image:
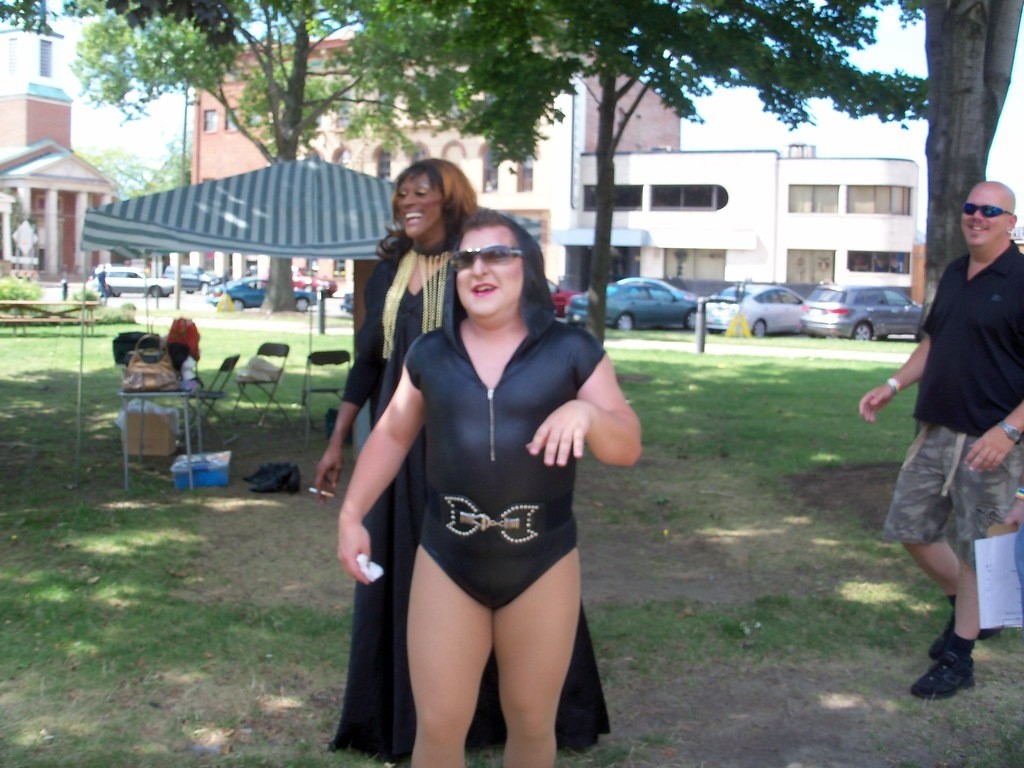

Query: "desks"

xmin=0 ymin=299 xmax=102 ymax=337
xmin=118 ymin=391 xmax=202 ymax=491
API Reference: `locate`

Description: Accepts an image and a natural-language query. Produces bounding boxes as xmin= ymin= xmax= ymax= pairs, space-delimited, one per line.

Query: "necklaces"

xmin=383 ymin=239 xmax=449 ymax=363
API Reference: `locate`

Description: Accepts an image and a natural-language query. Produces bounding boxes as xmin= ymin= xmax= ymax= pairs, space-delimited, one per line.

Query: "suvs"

xmin=86 ymin=264 xmax=175 ymax=299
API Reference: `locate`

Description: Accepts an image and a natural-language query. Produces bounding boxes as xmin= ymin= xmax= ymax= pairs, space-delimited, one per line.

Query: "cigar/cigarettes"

xmin=308 ymin=487 xmax=334 ymax=498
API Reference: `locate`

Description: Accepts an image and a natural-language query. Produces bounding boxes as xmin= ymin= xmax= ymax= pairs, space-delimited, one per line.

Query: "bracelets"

xmin=885 ymin=378 xmax=903 ymax=396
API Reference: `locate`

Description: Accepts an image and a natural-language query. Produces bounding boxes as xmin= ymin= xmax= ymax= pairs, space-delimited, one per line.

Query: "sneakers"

xmin=928 ymin=619 xmax=956 ymax=659
xmin=912 ymin=651 xmax=975 ymax=698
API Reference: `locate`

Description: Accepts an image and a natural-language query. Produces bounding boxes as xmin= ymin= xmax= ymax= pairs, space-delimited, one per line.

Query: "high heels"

xmin=244 ymin=461 xmax=300 ymax=494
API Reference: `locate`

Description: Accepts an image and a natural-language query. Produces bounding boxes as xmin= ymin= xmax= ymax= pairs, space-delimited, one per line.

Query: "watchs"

xmin=998 ymin=420 xmax=1021 ymax=445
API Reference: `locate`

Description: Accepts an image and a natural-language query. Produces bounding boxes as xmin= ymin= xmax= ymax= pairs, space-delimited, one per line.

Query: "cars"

xmin=562 ymin=277 xmax=699 ymax=332
xmin=161 ymin=264 xmax=205 ymax=294
xmin=542 ymin=277 xmax=583 ymax=316
xmin=701 ymin=284 xmax=807 ymax=338
xmin=798 ymin=280 xmax=923 ymax=340
xmin=205 ymin=266 xmax=354 ymax=318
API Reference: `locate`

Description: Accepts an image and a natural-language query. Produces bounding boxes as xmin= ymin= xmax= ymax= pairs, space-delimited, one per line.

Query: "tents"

xmin=75 ymin=158 xmax=542 ymax=491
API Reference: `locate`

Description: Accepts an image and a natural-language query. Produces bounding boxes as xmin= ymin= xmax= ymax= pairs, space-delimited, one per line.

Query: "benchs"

xmin=0 ymin=314 xmax=104 ymax=325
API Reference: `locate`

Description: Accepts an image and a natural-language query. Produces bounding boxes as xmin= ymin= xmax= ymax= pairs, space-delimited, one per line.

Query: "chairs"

xmin=299 ymin=350 xmax=351 ymax=452
xmin=180 ymin=354 xmax=241 ymax=446
xmin=231 ymin=342 xmax=292 ymax=427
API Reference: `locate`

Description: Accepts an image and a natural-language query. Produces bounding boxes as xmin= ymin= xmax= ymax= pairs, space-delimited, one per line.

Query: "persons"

xmin=314 ymin=159 xmax=610 ymax=768
xmin=98 ymin=266 xmax=109 ymax=306
xmin=858 ymin=182 xmax=1024 ymax=699
xmin=337 ymin=209 xmax=642 ymax=768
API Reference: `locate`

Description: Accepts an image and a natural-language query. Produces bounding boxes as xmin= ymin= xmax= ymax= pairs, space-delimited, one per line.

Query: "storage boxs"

xmin=325 ymin=407 xmax=353 ymax=444
xmin=169 ymin=449 xmax=231 ymax=490
xmin=119 ymin=410 xmax=178 ymax=457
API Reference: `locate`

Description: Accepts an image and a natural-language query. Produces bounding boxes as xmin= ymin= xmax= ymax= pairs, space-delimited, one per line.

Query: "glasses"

xmin=962 ymin=203 xmax=1012 ymax=219
xmin=448 ymin=244 xmax=523 ymax=272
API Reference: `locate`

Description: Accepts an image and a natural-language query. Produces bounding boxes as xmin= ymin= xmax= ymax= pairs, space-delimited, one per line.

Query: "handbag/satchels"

xmin=120 ymin=333 xmax=180 ymax=391
